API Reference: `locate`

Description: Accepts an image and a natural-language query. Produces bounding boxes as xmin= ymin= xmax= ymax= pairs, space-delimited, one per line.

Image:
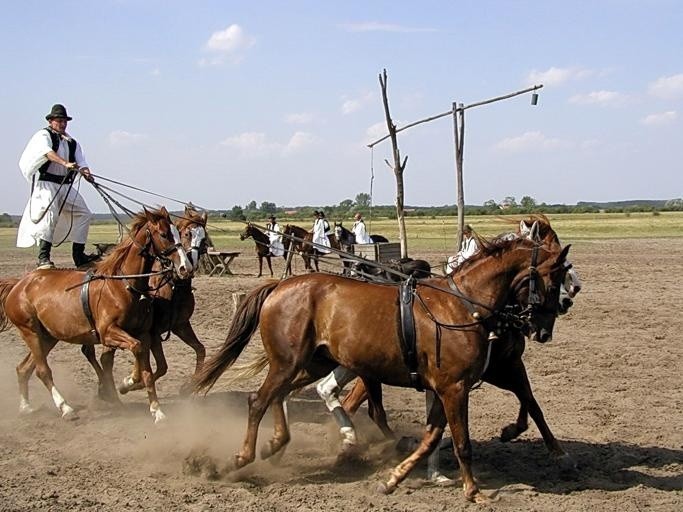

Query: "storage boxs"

xmin=351 ymin=242 xmax=400 ymax=262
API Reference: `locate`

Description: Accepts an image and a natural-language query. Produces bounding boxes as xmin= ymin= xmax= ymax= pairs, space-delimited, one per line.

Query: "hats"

xmin=45 ymin=104 xmax=73 ymax=121
xmin=268 ymin=214 xmax=276 ymax=219
xmin=313 ymin=211 xmax=324 ymax=216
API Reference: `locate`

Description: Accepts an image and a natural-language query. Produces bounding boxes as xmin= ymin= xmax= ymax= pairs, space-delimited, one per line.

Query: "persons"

xmin=445 ymin=224 xmax=482 ymax=275
xmin=350 ymin=213 xmax=375 ymax=244
xmin=263 ymin=214 xmax=285 ymax=257
xmin=306 ymin=211 xmax=333 ymax=256
xmin=16 ymin=104 xmax=101 ymax=270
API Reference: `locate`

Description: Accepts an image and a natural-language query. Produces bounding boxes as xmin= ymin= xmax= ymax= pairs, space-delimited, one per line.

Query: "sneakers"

xmin=76 ymin=254 xmax=100 ymax=268
xmin=38 ymin=258 xmax=54 ymax=266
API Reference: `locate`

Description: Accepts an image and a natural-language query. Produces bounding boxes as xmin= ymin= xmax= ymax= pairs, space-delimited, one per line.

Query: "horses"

xmin=338 ymin=210 xmax=580 ymax=470
xmin=239 ymin=222 xmax=292 ymax=278
xmin=333 ymin=220 xmax=389 ymax=275
xmin=0 ymin=203 xmax=194 ymax=426
xmin=183 ymin=233 xmax=572 ymax=506
xmin=212 ymin=219 xmax=573 ymax=485
xmin=80 ymin=205 xmax=209 ymax=404
xmin=281 ymin=223 xmax=348 ymax=274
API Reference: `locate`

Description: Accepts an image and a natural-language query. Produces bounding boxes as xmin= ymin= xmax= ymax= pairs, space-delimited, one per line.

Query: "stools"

xmin=207 ymin=251 xmax=240 ymax=277
